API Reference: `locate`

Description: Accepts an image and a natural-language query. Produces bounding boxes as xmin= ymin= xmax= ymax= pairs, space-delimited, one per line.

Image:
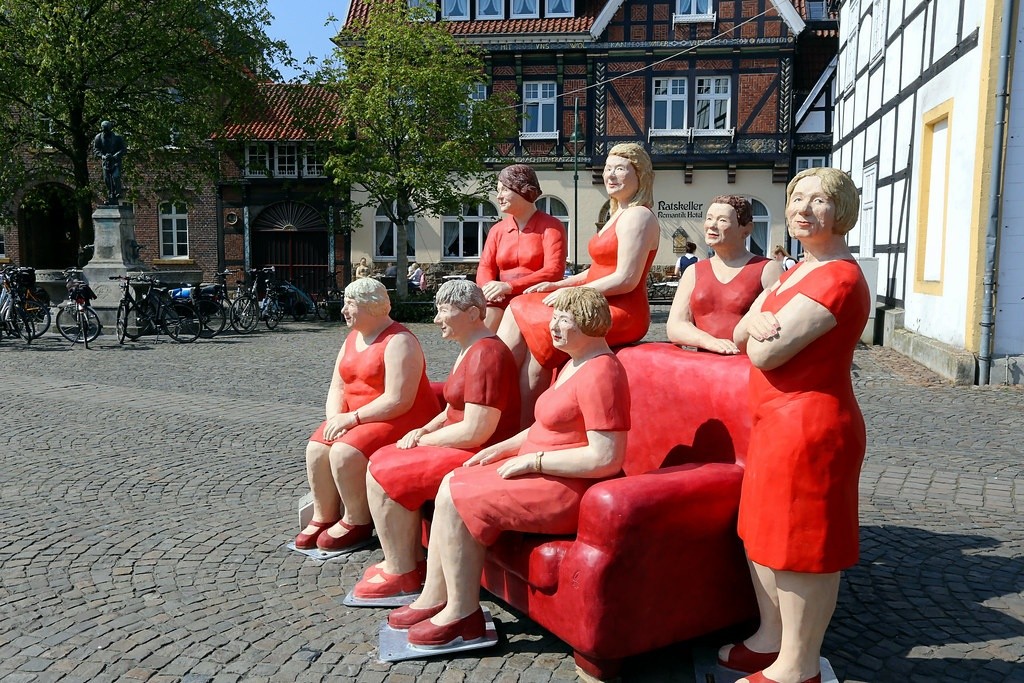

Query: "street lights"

xmin=567 ymin=96 xmax=588 ymax=276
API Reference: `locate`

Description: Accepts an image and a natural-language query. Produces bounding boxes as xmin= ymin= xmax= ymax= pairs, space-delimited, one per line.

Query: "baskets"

xmin=13 ymin=269 xmax=36 ymax=289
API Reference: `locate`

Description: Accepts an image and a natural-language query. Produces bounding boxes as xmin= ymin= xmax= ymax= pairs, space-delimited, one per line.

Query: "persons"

xmin=717 ymin=167 xmax=867 ymax=683
xmin=295 ymin=278 xmax=442 ymax=551
xmin=356 ymin=258 xmax=372 ymax=279
xmin=674 ymin=242 xmax=700 ymax=279
xmin=407 ymin=262 xmax=423 ymax=287
xmin=771 ymin=244 xmax=798 ymax=271
xmin=494 ymin=143 xmax=661 ymax=431
xmin=354 ymin=280 xmax=523 ymax=599
xmin=385 ymin=262 xmax=397 ymax=276
xmin=475 ymin=164 xmax=568 ymax=334
xmin=665 ymin=195 xmax=785 ymax=354
xmin=387 ymin=286 xmax=632 ymax=650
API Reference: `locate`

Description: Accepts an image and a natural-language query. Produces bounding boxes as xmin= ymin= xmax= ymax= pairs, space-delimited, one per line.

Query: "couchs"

xmin=419 ymin=341 xmax=760 ymax=683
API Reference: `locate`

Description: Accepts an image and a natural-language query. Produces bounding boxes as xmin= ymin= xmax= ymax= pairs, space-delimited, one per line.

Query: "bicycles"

xmin=0 ymin=263 xmax=52 ymax=346
xmin=56 ymin=266 xmax=104 ymax=348
xmin=109 ymin=266 xmax=340 ymax=343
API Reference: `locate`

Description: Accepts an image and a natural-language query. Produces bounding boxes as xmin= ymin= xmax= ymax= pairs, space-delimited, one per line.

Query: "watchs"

xmin=535 ymin=451 xmax=544 ymax=473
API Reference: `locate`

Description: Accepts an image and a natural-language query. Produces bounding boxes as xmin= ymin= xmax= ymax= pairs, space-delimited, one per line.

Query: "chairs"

xmin=410 ymin=272 xmax=426 ymax=294
xmin=381 ymin=276 xmax=396 ymax=291
xmin=649 ymin=274 xmax=667 ymax=300
